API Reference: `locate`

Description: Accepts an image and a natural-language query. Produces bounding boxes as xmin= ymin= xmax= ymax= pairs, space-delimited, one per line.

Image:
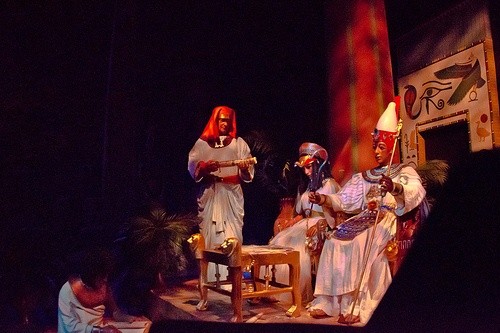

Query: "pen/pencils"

xmin=109 ymin=325 xmax=122 ymax=333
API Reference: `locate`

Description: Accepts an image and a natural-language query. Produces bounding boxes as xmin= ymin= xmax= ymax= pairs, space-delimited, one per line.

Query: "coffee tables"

xmin=188 ymin=234 xmax=301 ymax=323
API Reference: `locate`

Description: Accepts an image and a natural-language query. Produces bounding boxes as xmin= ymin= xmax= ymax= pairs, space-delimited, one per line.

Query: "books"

xmin=99 ymin=320 xmax=150 ymax=333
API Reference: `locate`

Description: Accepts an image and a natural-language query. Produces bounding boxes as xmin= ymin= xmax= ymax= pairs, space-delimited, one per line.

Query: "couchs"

xmin=274 ymin=197 xmax=429 ymax=278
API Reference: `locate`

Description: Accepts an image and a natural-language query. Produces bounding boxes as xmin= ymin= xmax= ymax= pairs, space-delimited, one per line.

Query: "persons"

xmin=257 ymin=141 xmax=342 ymax=297
xmin=58 ymin=247 xmax=153 ymax=333
xmin=307 ymin=129 xmax=427 ymax=324
xmin=187 ymin=104 xmax=258 ymax=289
